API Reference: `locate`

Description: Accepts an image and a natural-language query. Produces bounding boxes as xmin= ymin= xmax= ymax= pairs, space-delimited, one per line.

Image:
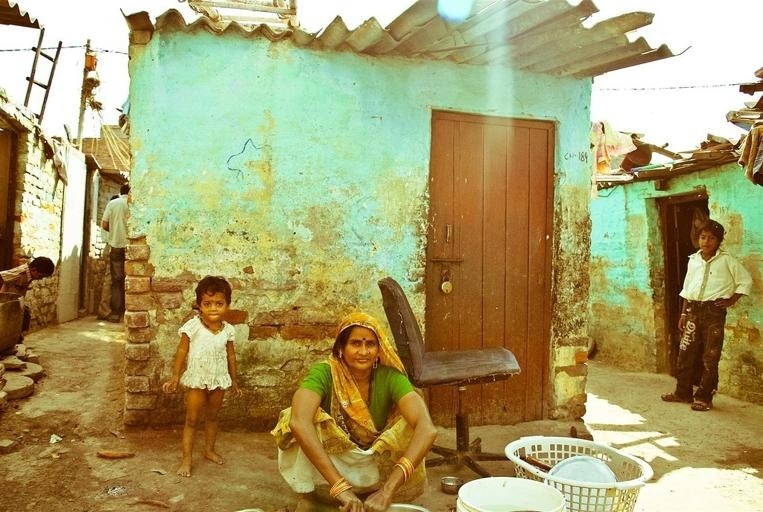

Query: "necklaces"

xmin=351 ymin=374 xmax=370 ymax=404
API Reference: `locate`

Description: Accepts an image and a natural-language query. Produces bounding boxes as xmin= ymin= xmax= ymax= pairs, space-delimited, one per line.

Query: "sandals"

xmin=691 ymin=397 xmax=713 ymax=411
xmin=661 ymin=391 xmax=694 ymax=402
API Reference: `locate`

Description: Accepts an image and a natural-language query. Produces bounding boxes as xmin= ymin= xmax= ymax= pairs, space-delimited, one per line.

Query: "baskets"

xmin=504 ymin=435 xmax=654 ymax=512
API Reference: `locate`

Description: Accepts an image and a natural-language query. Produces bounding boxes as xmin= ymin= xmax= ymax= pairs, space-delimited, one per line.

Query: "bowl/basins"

xmin=440 ymin=477 xmax=464 ymax=495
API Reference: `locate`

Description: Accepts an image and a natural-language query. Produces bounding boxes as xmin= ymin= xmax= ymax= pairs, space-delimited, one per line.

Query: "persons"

xmin=161 ymin=275 xmax=243 ymax=478
xmin=266 ymin=312 xmax=436 ymax=511
xmin=102 ymin=182 xmax=127 ymax=321
xmin=0 ymin=253 xmax=55 ymax=342
xmin=661 ymin=221 xmax=752 ymax=409
xmin=97 ymin=195 xmax=120 ymax=320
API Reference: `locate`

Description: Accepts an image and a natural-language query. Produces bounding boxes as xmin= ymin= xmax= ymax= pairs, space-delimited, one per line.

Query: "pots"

xmin=516 ymin=454 xmax=618 ymax=512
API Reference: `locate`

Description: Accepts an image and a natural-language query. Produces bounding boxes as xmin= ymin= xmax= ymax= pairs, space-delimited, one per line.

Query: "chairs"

xmin=376 ymin=275 xmax=523 ymax=484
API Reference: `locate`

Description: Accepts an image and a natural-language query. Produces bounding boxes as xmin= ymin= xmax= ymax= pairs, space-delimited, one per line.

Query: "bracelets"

xmin=329 ymin=475 xmax=352 ymax=498
xmin=394 ymin=455 xmax=414 ymax=484
xmin=680 ymin=313 xmax=688 ymax=317
